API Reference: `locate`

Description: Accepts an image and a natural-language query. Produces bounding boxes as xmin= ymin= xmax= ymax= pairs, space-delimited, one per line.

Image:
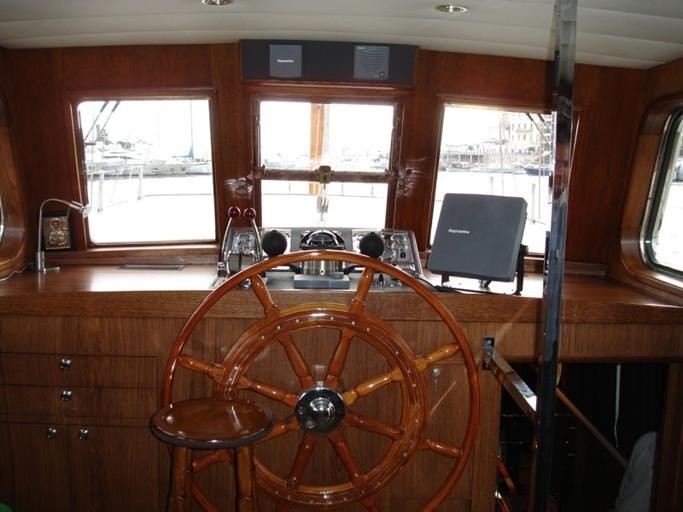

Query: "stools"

xmin=146 ymin=396 xmax=273 ymax=510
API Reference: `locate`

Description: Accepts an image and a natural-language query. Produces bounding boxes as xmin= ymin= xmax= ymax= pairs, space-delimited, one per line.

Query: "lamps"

xmin=35 ymin=199 xmax=93 ymax=274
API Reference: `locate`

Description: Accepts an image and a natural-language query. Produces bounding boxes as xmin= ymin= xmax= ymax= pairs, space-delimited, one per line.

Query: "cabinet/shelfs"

xmin=218 ymin=264 xmax=385 ymax=512
xmin=385 ymin=268 xmax=505 ymax=512
xmin=0 ymin=265 xmax=218 ymax=511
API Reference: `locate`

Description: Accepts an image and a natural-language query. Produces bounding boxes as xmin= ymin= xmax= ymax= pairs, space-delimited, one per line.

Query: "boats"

xmin=264 ymin=152 xmax=390 ymax=172
xmin=80 ymin=106 xmax=211 ymax=176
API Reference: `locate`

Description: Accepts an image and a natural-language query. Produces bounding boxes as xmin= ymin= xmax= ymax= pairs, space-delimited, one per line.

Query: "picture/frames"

xmin=38 ymin=209 xmax=74 ymax=252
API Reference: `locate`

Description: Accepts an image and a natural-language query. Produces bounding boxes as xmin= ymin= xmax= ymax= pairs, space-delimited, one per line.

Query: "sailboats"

xmin=438 ymin=139 xmax=551 ymax=176
